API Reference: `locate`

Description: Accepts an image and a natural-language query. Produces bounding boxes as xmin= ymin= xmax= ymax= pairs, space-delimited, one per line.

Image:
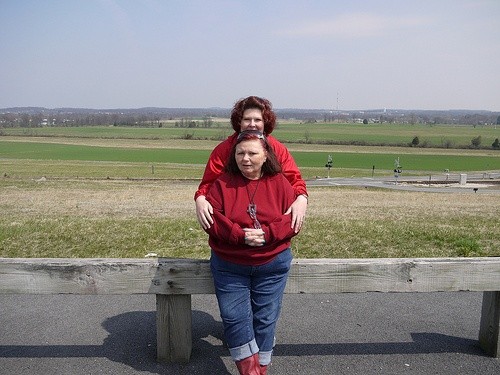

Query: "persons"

xmin=194 ymin=96 xmax=308 ymax=352
xmin=203 ymin=129 xmax=296 ymax=375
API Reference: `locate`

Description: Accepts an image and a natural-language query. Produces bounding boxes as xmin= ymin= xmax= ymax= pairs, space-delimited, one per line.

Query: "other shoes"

xmin=258 ymin=365 xmax=267 ymax=375
xmin=236 ymin=352 xmax=260 ymax=375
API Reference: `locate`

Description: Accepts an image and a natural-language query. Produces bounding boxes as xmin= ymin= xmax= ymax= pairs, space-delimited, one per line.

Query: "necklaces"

xmin=237 ymin=169 xmax=264 ymax=229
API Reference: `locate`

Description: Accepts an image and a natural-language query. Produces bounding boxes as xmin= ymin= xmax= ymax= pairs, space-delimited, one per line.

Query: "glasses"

xmin=246 ymin=208 xmax=261 ymax=229
